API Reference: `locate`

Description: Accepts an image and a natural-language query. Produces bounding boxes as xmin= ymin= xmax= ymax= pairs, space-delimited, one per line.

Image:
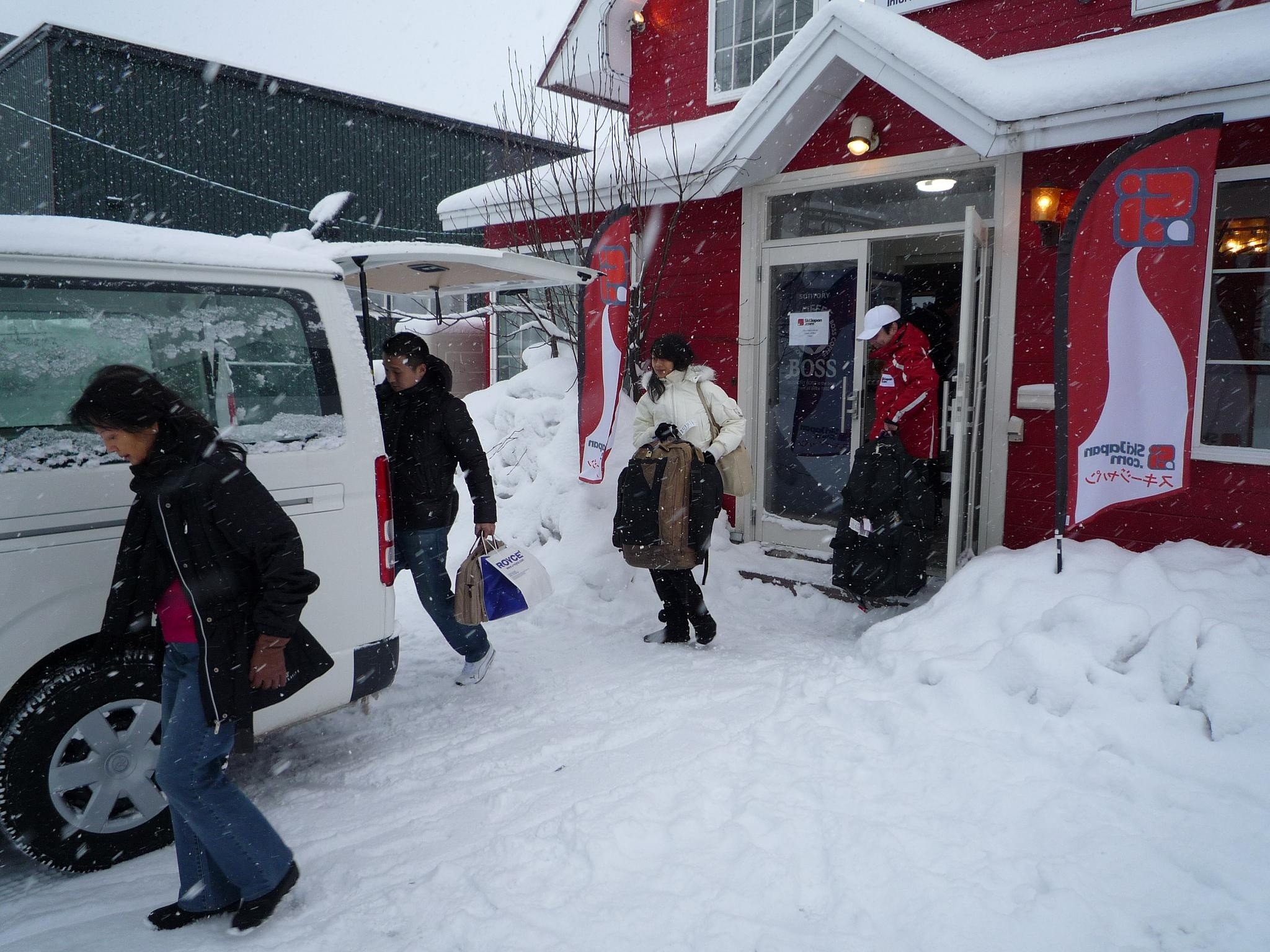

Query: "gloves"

xmin=654 ymin=422 xmax=679 ymax=442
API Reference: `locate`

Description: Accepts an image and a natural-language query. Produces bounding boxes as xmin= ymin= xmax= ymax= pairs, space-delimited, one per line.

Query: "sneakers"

xmin=455 ymin=641 xmax=495 ymax=686
xmin=149 ymin=892 xmax=240 ymax=930
xmin=692 ymin=614 xmax=717 ymax=644
xmin=231 ymin=861 xmax=299 ymax=933
xmin=643 ymin=624 xmax=690 ymax=643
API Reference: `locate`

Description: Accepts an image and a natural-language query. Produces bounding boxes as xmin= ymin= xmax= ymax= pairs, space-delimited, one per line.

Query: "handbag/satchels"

xmin=696 ymin=382 xmax=754 ymax=496
xmin=455 ymin=532 xmax=504 ymax=626
xmin=478 ymin=530 xmax=557 ymax=621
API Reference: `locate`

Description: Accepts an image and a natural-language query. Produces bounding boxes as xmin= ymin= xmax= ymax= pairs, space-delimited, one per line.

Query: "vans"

xmin=0 ymin=210 xmax=610 ymax=874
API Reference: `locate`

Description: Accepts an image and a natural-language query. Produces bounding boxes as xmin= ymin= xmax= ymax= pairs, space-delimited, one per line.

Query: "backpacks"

xmin=612 ymin=436 xmax=723 ymax=585
xmin=830 ymin=432 xmax=935 ymax=606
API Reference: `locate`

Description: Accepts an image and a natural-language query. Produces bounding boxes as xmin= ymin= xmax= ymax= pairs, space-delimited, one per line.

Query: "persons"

xmin=856 ymin=304 xmax=939 ymax=463
xmin=632 ymin=334 xmax=746 ymax=645
xmin=68 ymin=365 xmax=321 ymax=936
xmin=375 ymin=332 xmax=497 ymax=686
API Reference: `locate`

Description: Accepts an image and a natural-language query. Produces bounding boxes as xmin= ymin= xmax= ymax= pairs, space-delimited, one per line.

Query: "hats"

xmin=856 ymin=305 xmax=900 ymax=341
